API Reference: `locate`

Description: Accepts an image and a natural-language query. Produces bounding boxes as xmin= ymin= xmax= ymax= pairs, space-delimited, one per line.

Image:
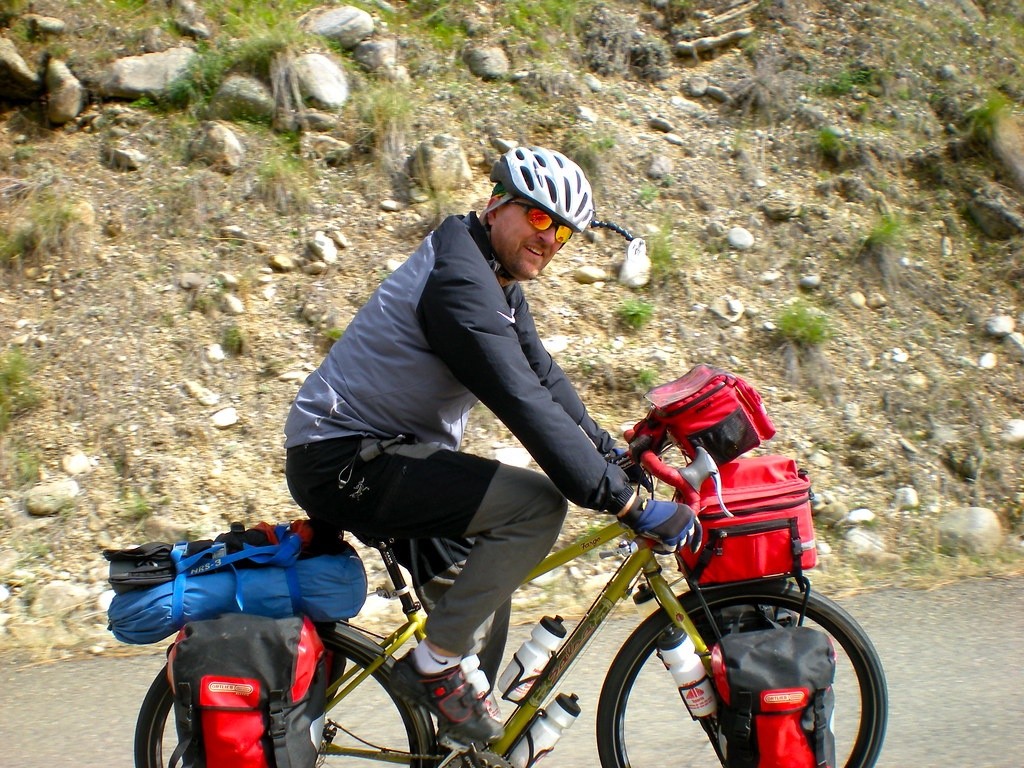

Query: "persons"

xmin=276 ymin=147 xmax=701 ymax=737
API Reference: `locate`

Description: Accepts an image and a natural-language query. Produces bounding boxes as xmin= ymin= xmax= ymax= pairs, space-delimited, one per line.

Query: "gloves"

xmin=618 ymin=495 xmax=703 ymax=554
xmin=612 ymin=448 xmax=652 ymax=493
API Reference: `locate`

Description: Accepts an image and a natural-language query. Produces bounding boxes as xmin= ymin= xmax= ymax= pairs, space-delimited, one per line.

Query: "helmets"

xmin=490 ymin=145 xmax=594 ymax=233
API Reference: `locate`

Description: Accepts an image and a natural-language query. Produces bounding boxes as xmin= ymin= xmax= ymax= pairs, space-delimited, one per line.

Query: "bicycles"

xmin=134 ymin=419 xmax=889 ymax=768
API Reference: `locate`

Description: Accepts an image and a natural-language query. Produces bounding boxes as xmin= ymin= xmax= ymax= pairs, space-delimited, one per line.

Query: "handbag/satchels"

xmin=648 ymin=363 xmax=776 ymax=468
xmin=673 ymin=455 xmax=816 ymax=588
xmin=712 ymin=626 xmax=836 ymax=768
xmin=100 ymin=517 xmax=367 ymax=768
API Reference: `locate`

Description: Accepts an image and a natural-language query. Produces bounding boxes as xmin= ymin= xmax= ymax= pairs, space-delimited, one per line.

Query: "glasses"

xmin=506 ymin=200 xmax=574 ymax=243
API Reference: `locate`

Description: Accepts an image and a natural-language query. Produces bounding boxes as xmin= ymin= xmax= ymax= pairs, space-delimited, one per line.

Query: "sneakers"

xmin=389 ymin=648 xmax=504 ymax=741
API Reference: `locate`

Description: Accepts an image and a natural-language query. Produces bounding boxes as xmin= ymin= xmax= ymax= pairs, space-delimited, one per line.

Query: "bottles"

xmin=508 ymin=693 xmax=581 ymax=768
xmin=460 ymin=654 xmax=502 ymax=723
xmin=657 ymin=625 xmax=718 ymax=717
xmin=497 ymin=614 xmax=567 ymax=700
